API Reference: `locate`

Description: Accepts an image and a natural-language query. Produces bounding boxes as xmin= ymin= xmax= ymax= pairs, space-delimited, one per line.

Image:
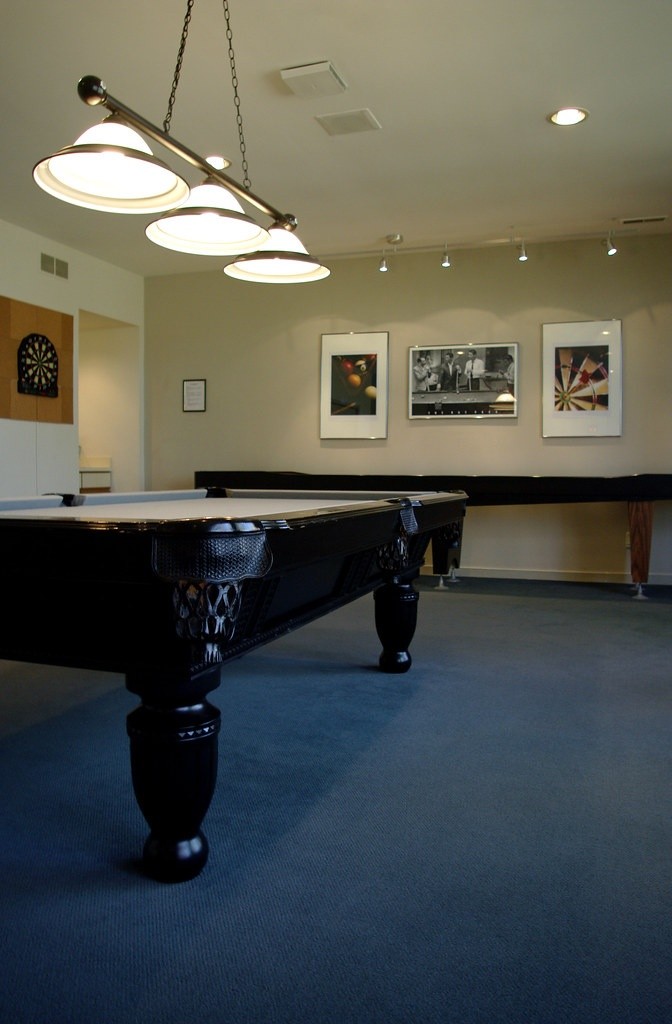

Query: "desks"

xmin=194 ymin=469 xmax=672 ymax=604
xmin=2 ymin=485 xmax=471 ymax=884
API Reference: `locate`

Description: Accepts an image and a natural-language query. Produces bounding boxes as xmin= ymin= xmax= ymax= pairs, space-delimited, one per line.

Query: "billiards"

xmin=413 ymin=394 xmax=482 ymax=404
xmin=341 ymin=361 xmax=354 ymax=372
xmin=356 ymin=360 xmax=369 ymax=374
xmin=348 ymin=373 xmax=362 ymax=386
xmin=366 ymin=386 xmax=376 ymax=398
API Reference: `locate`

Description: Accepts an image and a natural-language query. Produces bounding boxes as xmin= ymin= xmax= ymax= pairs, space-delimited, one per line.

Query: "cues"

xmin=456 ymin=371 xmax=460 ymax=389
xmin=468 ymin=373 xmax=472 ymax=389
xmin=423 ymin=376 xmax=432 ymax=390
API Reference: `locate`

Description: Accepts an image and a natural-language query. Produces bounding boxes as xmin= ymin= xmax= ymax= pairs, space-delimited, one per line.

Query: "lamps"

xmin=441 ymin=242 xmax=450 ymax=268
xmin=379 ymin=249 xmax=388 ymax=273
xmin=604 ymin=232 xmax=618 ymax=256
xmin=31 ymin=0 xmax=330 ymax=283
xmin=519 ymin=238 xmax=527 ymax=262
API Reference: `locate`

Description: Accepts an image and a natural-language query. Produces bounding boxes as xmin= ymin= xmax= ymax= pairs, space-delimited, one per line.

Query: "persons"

xmin=414 ymin=358 xmax=432 ymax=392
xmin=499 ymin=355 xmax=515 ymax=395
xmin=437 ymin=353 xmax=461 ymax=392
xmin=464 ymin=350 xmax=485 ymax=391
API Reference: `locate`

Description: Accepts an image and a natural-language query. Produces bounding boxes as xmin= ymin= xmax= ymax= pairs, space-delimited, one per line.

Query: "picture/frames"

xmin=182 ymin=379 xmax=206 ymax=413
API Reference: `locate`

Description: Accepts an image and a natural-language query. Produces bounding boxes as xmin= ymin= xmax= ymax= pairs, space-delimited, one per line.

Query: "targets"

xmin=17 ymin=331 xmax=60 ymax=398
xmin=555 ymin=346 xmax=610 ymax=413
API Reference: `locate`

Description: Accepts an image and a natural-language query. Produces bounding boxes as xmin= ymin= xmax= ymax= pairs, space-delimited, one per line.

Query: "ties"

xmin=470 ymin=364 xmax=473 ymax=381
